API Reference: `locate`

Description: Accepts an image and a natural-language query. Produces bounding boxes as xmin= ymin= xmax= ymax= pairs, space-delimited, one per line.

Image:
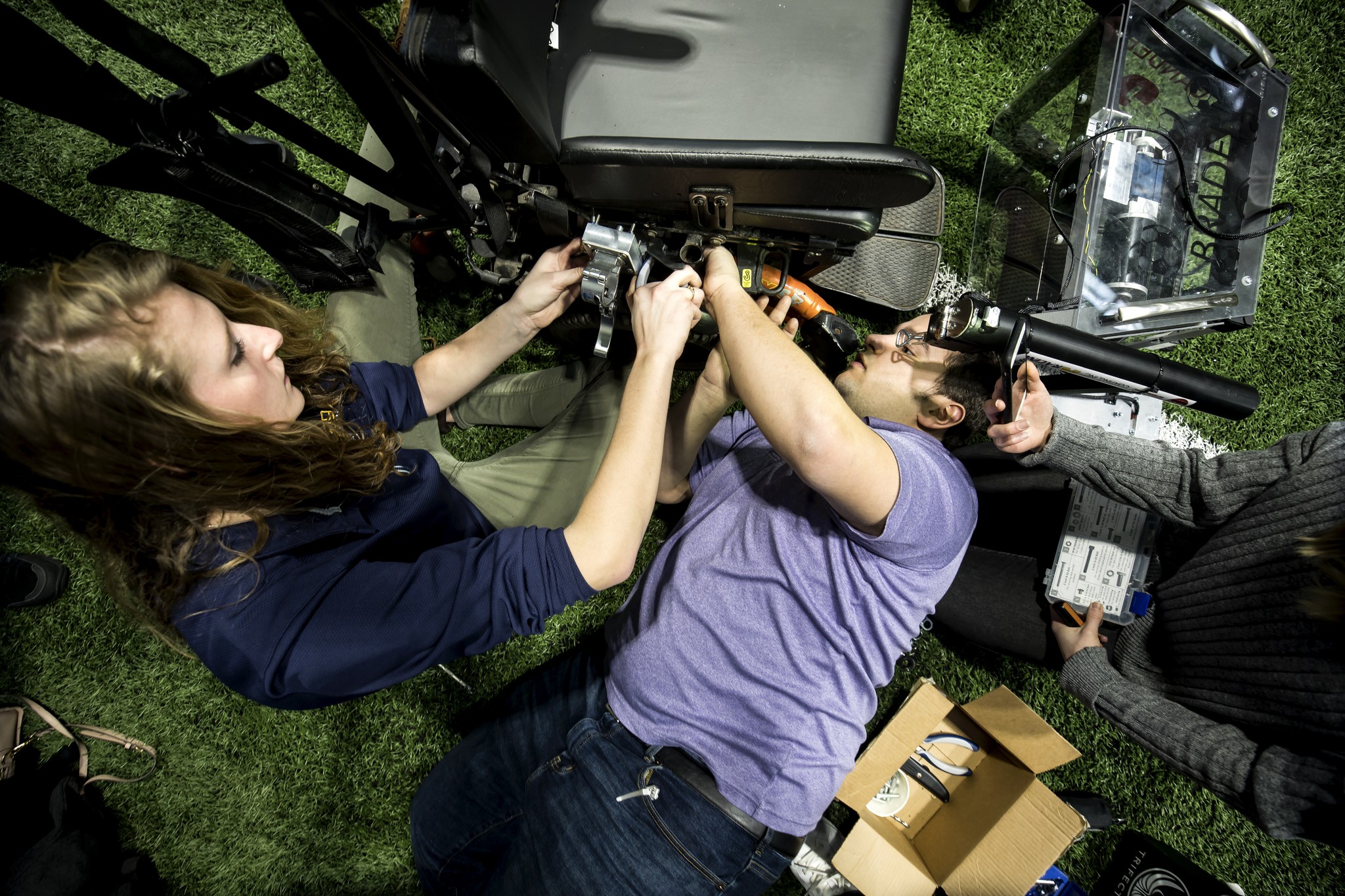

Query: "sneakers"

xmin=0 ymin=549 xmax=71 ymax=613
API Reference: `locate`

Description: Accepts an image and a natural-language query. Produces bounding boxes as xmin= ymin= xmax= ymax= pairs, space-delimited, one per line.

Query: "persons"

xmin=984 ymin=354 xmax=1345 ymax=870
xmin=402 ymin=233 xmax=990 ymax=896
xmin=0 ymin=227 xmax=712 ymax=722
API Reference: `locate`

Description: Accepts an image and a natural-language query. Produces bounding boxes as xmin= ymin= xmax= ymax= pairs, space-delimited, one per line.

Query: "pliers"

xmin=914 ymin=732 xmax=979 ymax=777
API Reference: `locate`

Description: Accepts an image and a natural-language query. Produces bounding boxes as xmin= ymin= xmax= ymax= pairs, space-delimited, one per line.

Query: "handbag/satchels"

xmin=0 ymin=705 xmax=24 ymax=781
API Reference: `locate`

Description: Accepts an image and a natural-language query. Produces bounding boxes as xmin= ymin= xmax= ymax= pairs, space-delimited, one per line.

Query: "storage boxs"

xmin=830 ymin=676 xmax=1091 ymax=895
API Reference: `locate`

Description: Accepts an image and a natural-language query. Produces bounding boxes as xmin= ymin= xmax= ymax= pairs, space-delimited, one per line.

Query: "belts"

xmin=611 ymin=705 xmax=806 ymax=859
